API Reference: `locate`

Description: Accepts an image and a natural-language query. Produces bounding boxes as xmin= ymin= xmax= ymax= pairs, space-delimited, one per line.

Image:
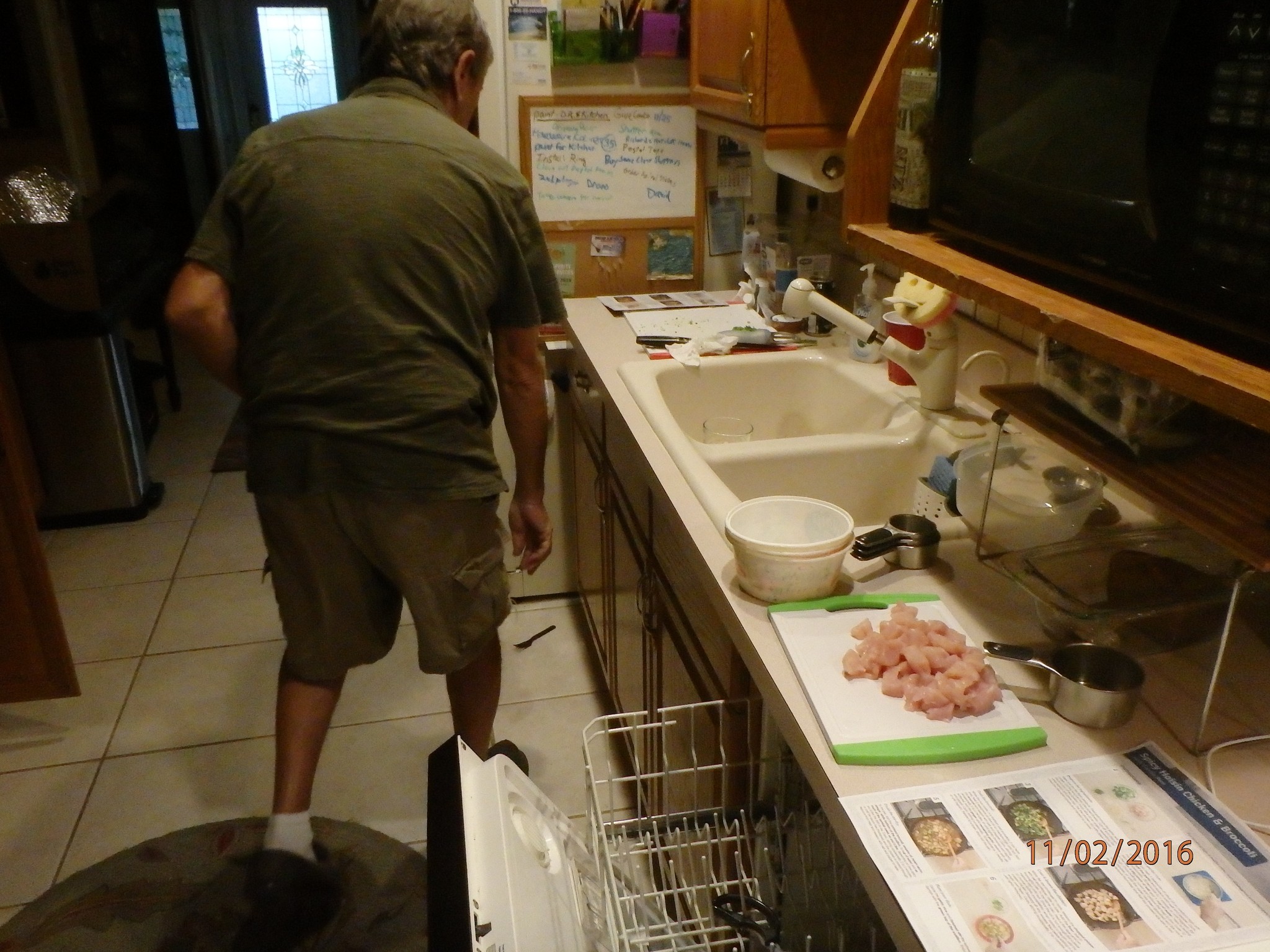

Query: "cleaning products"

xmin=849 ymin=261 xmax=885 ymax=363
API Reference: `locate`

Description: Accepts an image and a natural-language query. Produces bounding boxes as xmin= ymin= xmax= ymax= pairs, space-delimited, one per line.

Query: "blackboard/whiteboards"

xmin=519 ymin=89 xmax=704 ymax=235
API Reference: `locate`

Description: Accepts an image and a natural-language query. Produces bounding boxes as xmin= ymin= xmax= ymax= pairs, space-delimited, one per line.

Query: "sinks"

xmin=617 ymin=347 xmax=957 ymax=470
xmin=686 ymin=432 xmax=972 ymax=554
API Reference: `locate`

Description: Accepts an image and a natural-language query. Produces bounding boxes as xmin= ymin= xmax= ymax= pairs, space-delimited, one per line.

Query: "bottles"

xmin=885 ymin=0 xmax=945 ymax=233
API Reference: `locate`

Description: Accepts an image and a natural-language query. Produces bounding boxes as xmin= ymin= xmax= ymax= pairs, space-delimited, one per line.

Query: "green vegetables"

xmin=1009 ymin=803 xmax=1046 ymax=837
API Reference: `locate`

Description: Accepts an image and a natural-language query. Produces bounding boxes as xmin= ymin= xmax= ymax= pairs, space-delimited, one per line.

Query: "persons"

xmin=164 ymin=0 xmax=568 ymax=926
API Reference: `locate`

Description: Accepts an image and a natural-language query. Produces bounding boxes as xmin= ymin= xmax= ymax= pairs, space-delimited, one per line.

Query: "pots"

xmin=982 ymin=642 xmax=1147 ymax=729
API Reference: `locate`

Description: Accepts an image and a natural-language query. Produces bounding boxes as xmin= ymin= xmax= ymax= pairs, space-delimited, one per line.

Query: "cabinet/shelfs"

xmin=687 ymin=0 xmax=893 ymax=150
xmin=568 ymin=358 xmax=760 ymax=952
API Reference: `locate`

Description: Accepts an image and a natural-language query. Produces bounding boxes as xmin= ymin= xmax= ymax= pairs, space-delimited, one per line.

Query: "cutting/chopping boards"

xmin=621 ymin=300 xmax=798 ymax=359
xmin=767 ymin=591 xmax=1049 ymax=766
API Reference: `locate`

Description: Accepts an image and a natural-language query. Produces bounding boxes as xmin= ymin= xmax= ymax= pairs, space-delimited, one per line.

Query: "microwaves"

xmin=935 ymin=1 xmax=1270 ymax=363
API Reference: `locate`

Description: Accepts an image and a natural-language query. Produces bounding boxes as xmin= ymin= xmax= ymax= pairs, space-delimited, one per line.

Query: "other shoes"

xmin=487 ymin=738 xmax=529 ymax=777
xmin=246 ymin=839 xmax=340 ymax=924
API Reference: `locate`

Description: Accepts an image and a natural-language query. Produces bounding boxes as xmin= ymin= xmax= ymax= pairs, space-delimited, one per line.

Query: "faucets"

xmin=961 ymin=351 xmax=1011 ymax=384
xmin=780 ymin=272 xmax=961 ymax=416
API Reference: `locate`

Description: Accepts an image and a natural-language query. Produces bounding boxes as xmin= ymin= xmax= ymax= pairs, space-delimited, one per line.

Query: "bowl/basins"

xmin=724 ymin=495 xmax=856 ymax=604
xmin=953 ymin=433 xmax=1106 ymax=551
xmin=999 ymin=521 xmax=1247 ymax=656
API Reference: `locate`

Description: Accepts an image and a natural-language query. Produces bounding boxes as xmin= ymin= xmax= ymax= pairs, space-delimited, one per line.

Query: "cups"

xmin=883 ymin=311 xmax=926 ymax=386
xmin=600 ymin=26 xmax=638 ymax=62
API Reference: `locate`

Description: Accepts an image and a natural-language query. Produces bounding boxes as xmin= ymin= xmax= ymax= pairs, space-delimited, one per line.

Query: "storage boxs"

xmin=553 ymin=30 xmax=637 ymax=63
xmin=641 ymin=12 xmax=680 ymax=58
